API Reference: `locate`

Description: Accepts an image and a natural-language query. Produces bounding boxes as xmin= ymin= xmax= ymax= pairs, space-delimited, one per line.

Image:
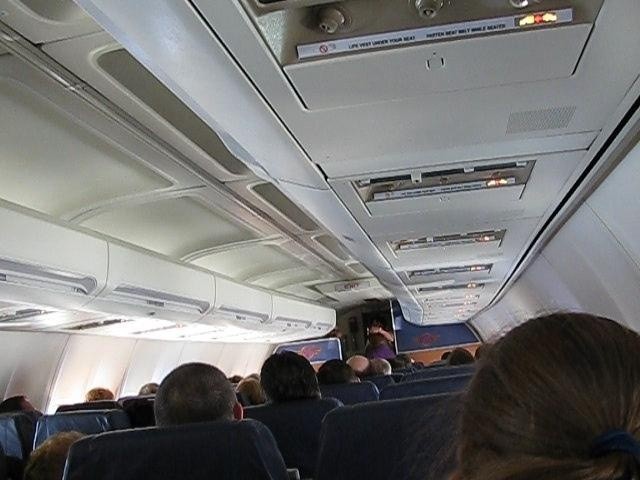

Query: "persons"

xmin=86 ymin=388 xmax=115 ymax=401
xmin=451 ymin=312 xmax=638 ymax=480
xmin=23 ymin=430 xmax=87 ymax=479
xmin=441 ymin=344 xmax=492 ymax=365
xmin=153 ymin=362 xmax=243 ymax=426
xmin=0 ymin=395 xmax=35 ymax=411
xmin=140 ymin=383 xmax=160 ymax=395
xmin=260 ymin=351 xmax=322 ymax=403
xmin=230 ymin=367 xmax=264 ymax=406
xmin=319 ymin=320 xmax=417 ymax=384
